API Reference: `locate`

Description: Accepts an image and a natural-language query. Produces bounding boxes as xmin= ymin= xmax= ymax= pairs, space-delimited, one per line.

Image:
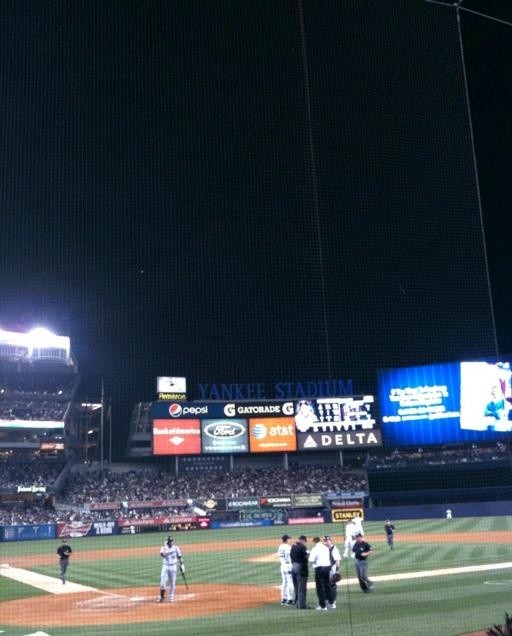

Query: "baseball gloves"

xmin=332 ymin=573 xmax=341 ymax=582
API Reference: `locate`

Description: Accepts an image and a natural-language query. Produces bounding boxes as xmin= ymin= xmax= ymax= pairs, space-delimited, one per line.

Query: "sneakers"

xmin=281 ymin=597 xmax=337 ymax=611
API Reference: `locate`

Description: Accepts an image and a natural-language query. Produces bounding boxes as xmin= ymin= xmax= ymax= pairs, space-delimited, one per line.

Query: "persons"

xmin=446 ymin=507 xmax=453 ymax=518
xmin=352 ymin=514 xmax=365 ymax=535
xmin=369 ymin=444 xmax=512 ymax=466
xmin=65 ymin=467 xmax=364 ymax=521
xmin=343 ymin=519 xmax=359 ymax=557
xmin=353 ymin=532 xmax=373 ymax=591
xmin=0 ymin=386 xmax=63 ymax=523
xmin=55 ymin=537 xmax=72 ymax=582
xmin=278 ymin=534 xmax=342 ymax=611
xmin=158 ymin=534 xmax=184 ymax=602
xmin=385 ymin=519 xmax=396 ymax=551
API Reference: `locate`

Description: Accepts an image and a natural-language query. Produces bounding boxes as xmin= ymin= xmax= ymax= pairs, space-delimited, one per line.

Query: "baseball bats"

xmin=178 ymin=567 xmax=189 ymax=588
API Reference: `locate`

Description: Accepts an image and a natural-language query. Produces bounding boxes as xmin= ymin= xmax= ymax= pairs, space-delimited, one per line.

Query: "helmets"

xmin=166 ymin=536 xmax=173 ymax=541
xmin=282 ymin=531 xmax=363 ymax=542
